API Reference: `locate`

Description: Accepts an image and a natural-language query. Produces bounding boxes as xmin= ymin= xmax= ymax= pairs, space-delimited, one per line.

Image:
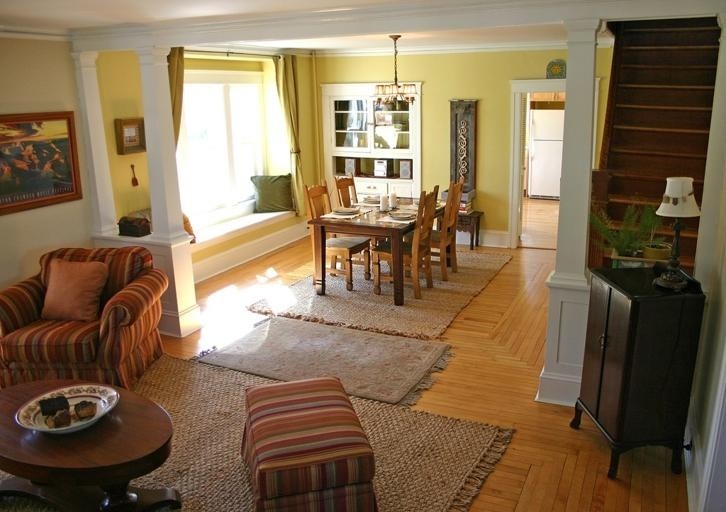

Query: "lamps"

xmin=376 ymin=34 xmax=416 ymax=108
xmin=651 ymin=177 xmax=701 ymax=293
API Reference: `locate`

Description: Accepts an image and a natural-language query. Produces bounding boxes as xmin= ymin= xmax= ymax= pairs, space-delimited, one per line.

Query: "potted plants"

xmin=586 ymin=204 xmax=673 ymax=268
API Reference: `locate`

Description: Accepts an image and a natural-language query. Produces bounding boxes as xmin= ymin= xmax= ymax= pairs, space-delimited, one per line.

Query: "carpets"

xmin=191 ymin=315 xmax=455 ymax=407
xmin=246 ymin=246 xmax=514 ymax=339
xmin=0 ymin=352 xmax=516 ymax=512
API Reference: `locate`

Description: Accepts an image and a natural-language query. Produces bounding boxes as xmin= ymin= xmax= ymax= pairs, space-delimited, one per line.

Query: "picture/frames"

xmin=115 ymin=117 xmax=146 ymax=155
xmin=0 ymin=111 xmax=83 ymax=216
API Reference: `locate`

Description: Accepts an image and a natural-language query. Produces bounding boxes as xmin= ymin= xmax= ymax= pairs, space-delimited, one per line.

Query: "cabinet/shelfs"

xmin=569 ymin=266 xmax=707 ymax=480
xmin=320 ymin=81 xmax=422 ymax=198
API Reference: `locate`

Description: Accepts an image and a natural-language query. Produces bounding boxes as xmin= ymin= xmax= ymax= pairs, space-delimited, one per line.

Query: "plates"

xmin=16 ymin=384 xmax=121 ymax=434
xmin=331 ymin=201 xmax=442 ymax=220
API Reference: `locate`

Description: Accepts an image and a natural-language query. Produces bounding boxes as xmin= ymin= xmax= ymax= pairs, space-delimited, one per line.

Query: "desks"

xmin=437 ymin=211 xmax=484 ymax=250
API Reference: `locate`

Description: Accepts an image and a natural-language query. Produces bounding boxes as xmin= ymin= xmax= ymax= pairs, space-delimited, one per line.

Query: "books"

xmin=373 ymin=159 xmax=394 ymax=178
xmin=344 ymin=158 xmax=361 ymax=177
xmin=442 ymin=200 xmax=475 ymax=214
xmin=400 ymin=160 xmax=411 ymax=179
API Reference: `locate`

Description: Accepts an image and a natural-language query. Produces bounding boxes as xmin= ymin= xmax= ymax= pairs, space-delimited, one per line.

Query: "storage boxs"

xmin=117 ymin=216 xmax=151 ymax=237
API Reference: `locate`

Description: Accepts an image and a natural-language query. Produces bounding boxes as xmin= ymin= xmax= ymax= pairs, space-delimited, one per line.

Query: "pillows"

xmin=250 ymin=173 xmax=294 ymax=212
xmin=41 ymin=257 xmax=110 ymax=322
xmin=184 ymin=212 xmax=196 ymax=244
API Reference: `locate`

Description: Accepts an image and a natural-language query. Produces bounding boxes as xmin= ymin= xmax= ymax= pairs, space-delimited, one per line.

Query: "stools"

xmin=241 ymin=375 xmax=378 ymax=512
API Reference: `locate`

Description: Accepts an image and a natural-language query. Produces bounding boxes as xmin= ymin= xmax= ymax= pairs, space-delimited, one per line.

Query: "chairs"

xmin=0 ymin=247 xmax=169 ymax=392
xmin=333 ymin=174 xmax=387 ymax=274
xmin=303 ymin=180 xmax=371 ymax=291
xmin=372 ymin=184 xmax=440 ymax=299
xmin=404 ymin=179 xmax=464 ymax=281
